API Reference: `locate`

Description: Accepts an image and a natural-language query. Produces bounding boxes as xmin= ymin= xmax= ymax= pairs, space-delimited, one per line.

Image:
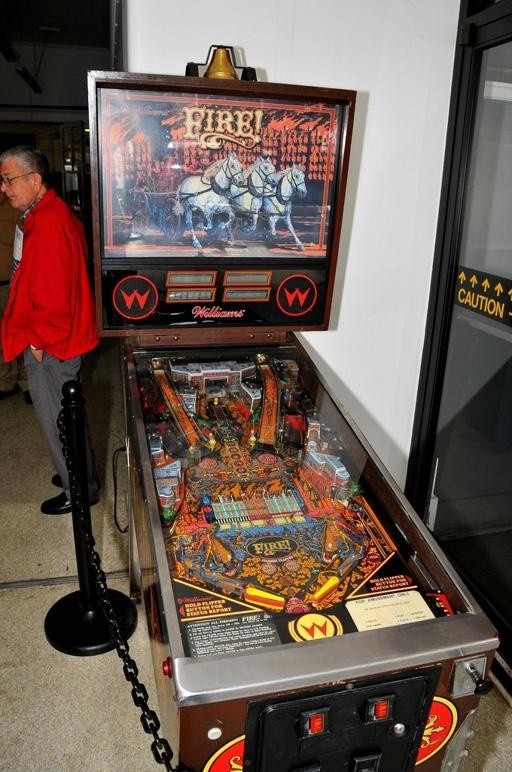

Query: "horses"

xmin=171 ymin=151 xmax=247 ymax=250
xmin=261 ymin=162 xmax=311 ymax=252
xmin=218 ymin=155 xmax=276 ymax=247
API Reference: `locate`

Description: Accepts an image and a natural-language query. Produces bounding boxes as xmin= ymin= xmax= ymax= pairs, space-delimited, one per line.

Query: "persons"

xmin=0 ymin=180 xmax=35 ymax=405
xmin=0 ymin=143 xmax=101 ymax=516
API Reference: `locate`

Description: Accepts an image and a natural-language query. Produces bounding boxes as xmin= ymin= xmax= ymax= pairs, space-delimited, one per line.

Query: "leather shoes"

xmin=40 ymin=488 xmax=100 ymax=515
xmin=51 ymin=473 xmax=101 ymax=492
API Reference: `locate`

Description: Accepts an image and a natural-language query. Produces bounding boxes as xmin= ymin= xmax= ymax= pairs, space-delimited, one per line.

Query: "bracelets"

xmin=30 ymin=345 xmax=45 ymax=351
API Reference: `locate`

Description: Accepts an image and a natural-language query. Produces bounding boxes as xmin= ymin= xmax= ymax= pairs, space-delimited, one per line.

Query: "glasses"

xmin=0 ymin=171 xmax=37 ymax=184
